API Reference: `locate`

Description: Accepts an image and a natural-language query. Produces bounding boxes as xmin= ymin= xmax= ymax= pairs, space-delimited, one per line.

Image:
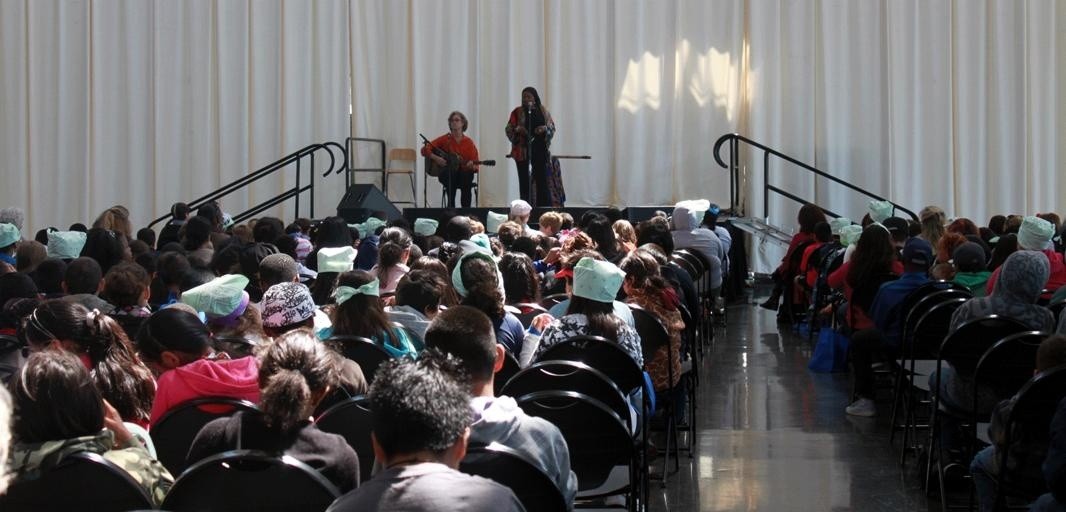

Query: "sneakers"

xmin=845 ymin=398 xmax=877 ymax=417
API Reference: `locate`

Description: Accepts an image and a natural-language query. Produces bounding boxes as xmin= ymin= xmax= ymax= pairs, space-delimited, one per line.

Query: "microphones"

xmin=528 ymin=102 xmax=533 ymax=115
xmin=446 ymin=133 xmax=452 ymax=138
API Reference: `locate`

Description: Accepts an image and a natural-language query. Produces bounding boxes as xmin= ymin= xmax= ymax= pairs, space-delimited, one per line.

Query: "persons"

xmin=4 ymin=196 xmax=1066 ymax=511
xmin=504 ymin=86 xmax=556 ymax=207
xmin=419 ymin=111 xmax=479 ymax=208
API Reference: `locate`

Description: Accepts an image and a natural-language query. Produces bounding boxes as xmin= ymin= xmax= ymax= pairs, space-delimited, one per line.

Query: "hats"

xmin=638 ymin=224 xmax=673 ymax=254
xmin=241 ymin=245 xmax=268 ymax=267
xmin=883 ymin=217 xmax=909 ymax=231
xmin=903 ymin=237 xmax=934 ymax=264
xmin=261 ymin=281 xmax=315 ymax=328
xmin=17 ymin=242 xmax=48 ymax=276
xmin=511 ymin=200 xmax=533 ymax=216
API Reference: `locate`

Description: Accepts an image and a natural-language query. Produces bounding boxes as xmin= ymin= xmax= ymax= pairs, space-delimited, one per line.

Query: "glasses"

xmin=449 ymin=118 xmax=462 ymax=121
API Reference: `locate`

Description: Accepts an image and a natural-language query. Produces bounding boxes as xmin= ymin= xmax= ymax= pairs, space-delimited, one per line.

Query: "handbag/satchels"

xmin=808 ymin=327 xmax=850 ymax=373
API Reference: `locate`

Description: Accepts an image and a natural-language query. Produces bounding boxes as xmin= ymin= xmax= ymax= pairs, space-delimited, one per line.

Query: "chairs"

xmin=441 ymin=173 xmax=479 ymax=208
xmin=384 ymin=147 xmax=418 ymax=209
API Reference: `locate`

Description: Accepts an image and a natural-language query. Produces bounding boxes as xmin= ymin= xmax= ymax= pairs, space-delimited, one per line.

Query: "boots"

xmin=760 ymin=287 xmax=782 ymax=311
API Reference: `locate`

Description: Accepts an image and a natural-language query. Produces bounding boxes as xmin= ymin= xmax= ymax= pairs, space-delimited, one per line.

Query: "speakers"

xmin=337 ymin=183 xmax=401 ymax=221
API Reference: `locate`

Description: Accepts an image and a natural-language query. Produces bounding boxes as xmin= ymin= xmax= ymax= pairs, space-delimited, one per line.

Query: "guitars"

xmin=424 ymin=147 xmax=496 ymax=178
